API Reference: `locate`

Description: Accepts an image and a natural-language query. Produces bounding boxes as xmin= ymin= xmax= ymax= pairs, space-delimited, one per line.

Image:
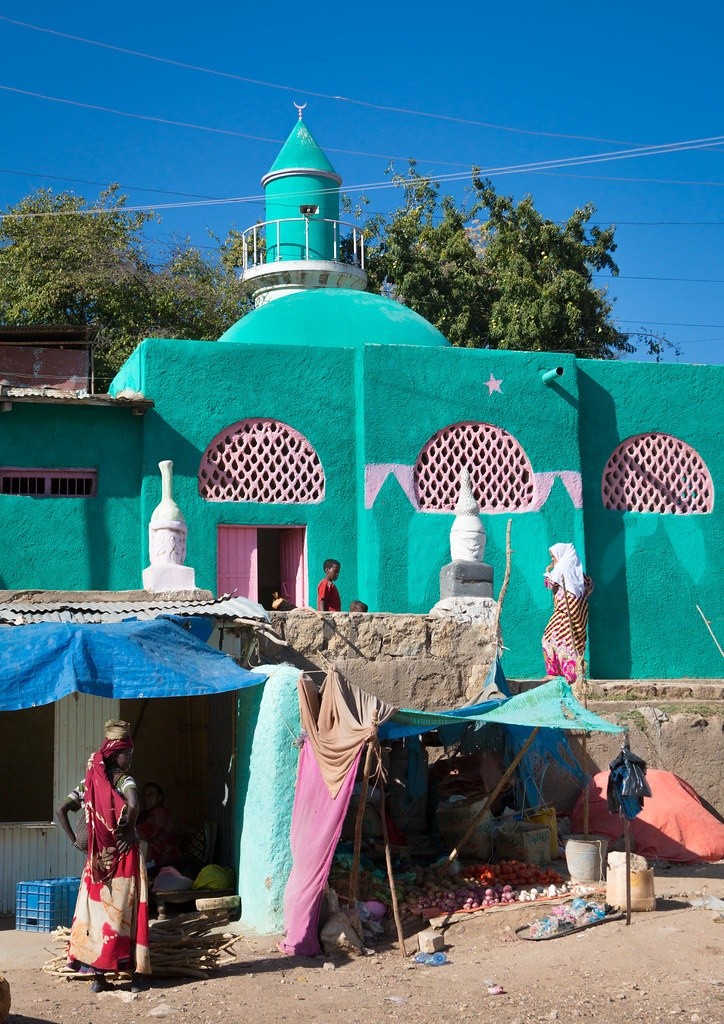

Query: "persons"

xmin=56 ymin=720 xmax=154 ymax=991
xmin=135 ymin=783 xmax=181 ymax=877
xmin=318 ymin=559 xmax=342 ymax=611
xmin=541 ymin=543 xmax=595 ymax=685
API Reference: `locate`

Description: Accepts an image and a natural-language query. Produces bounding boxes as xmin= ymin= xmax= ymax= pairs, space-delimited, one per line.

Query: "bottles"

xmin=414 ymin=952 xmax=445 ymax=966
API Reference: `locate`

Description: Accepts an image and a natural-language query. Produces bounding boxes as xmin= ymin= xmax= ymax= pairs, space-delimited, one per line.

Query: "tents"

xmin=1 ymin=592 xmax=631 ymax=958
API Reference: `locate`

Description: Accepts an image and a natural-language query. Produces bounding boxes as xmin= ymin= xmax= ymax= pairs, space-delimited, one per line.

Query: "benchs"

xmin=136 ymin=819 xmax=234 ymax=920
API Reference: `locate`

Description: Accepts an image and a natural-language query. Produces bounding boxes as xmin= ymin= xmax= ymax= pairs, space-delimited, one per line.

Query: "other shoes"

xmin=92 ymin=982 xmax=115 ymax=992
xmin=542 ymin=675 xmax=558 ymax=681
xmin=78 ymin=964 xmax=103 ymax=977
xmin=67 ymin=961 xmax=80 ymax=969
xmin=131 ymin=977 xmax=161 ymax=993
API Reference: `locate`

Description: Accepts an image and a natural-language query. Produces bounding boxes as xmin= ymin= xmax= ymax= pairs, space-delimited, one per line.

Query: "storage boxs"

xmin=436 ymin=793 xmax=552 ymax=867
xmin=15 ymin=877 xmax=82 ymax=933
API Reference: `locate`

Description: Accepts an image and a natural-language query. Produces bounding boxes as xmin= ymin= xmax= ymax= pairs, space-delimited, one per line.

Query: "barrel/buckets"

xmin=563 ymin=835 xmax=610 ymax=883
